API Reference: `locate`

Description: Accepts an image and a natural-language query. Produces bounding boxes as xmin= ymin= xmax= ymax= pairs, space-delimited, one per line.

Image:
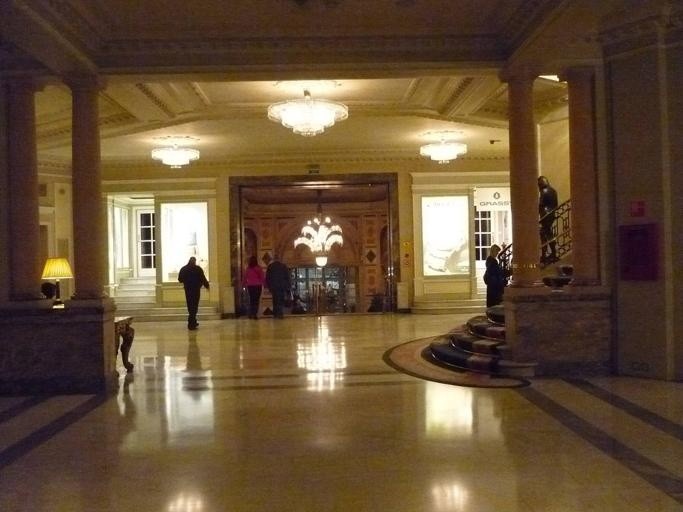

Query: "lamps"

xmin=151 ymin=136 xmax=201 ymax=169
xmin=267 ymin=80 xmax=348 ymax=137
xmin=41 ymin=257 xmax=74 ymax=309
xmin=419 ymin=130 xmax=467 ymax=165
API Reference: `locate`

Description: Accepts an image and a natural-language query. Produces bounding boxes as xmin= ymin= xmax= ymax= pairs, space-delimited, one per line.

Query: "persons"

xmin=291 ymin=298 xmax=305 ymax=313
xmin=242 ymin=256 xmax=266 ymax=319
xmin=484 ymin=245 xmax=510 ymax=307
xmin=264 ymin=254 xmax=292 ymax=319
xmin=538 ymin=176 xmax=559 ymax=261
xmin=178 ymin=256 xmax=210 ymax=330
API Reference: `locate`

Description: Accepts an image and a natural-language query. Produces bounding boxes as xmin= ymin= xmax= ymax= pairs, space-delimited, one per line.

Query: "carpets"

xmin=382 ymin=336 xmax=532 ymax=389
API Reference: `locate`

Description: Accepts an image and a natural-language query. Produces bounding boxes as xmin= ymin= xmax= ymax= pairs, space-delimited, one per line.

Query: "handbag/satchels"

xmin=285 ymin=295 xmax=292 ymax=308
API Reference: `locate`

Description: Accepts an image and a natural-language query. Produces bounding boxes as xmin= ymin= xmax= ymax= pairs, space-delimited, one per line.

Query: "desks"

xmin=114 ymin=316 xmax=135 ymax=377
